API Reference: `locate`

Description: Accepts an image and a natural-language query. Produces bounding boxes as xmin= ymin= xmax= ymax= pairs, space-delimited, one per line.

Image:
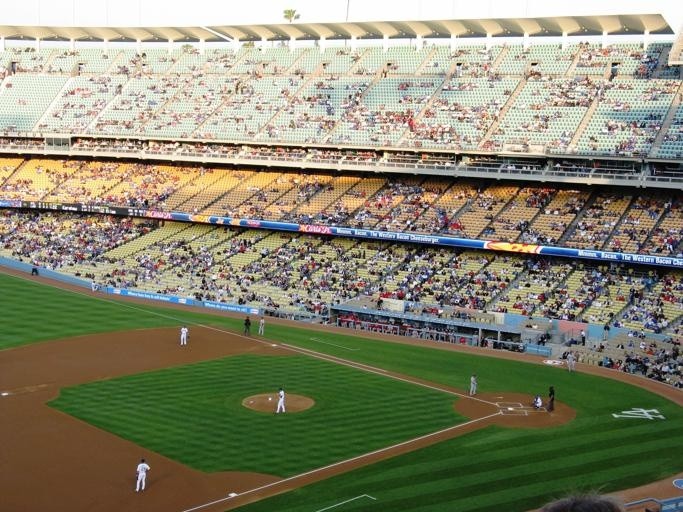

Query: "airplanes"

xmin=187 ymin=335 xmax=191 ymax=338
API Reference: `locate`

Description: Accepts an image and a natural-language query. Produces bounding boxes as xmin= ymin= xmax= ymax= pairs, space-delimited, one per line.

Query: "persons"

xmin=0 ymin=156 xmax=683 ymax=259
xmin=245 ymin=316 xmax=251 ymax=336
xmin=0 ymin=209 xmax=681 ymax=385
xmin=134 ymin=458 xmax=151 ymax=493
xmin=258 ymin=317 xmax=264 ymax=337
xmin=536 ymin=494 xmax=623 ymax=512
xmin=547 ymin=386 xmax=556 ymax=413
xmin=180 ymin=326 xmax=188 ymax=345
xmin=532 ymin=394 xmax=542 ymax=410
xmin=2 ymin=40 xmax=682 ymax=187
xmin=468 ymin=373 xmax=480 ymax=396
xmin=275 ymin=388 xmax=287 ymax=413
